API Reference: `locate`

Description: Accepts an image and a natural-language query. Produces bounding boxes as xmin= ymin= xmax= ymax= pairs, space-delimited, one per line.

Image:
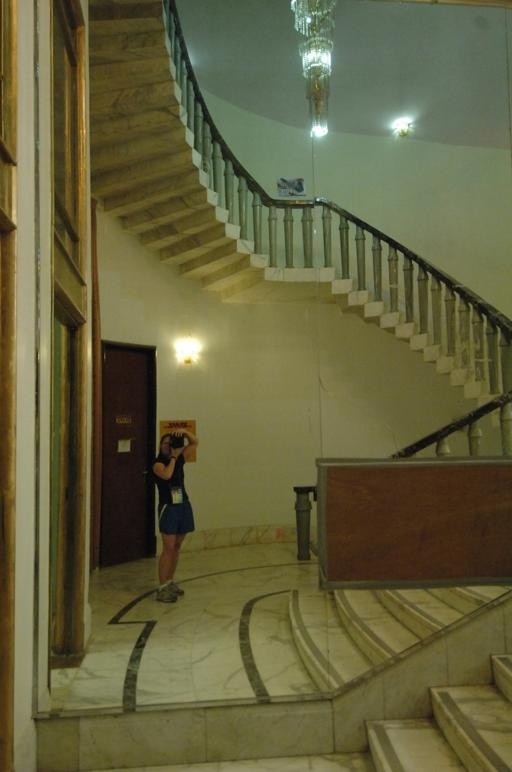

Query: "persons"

xmin=152 ymin=428 xmax=199 ymax=604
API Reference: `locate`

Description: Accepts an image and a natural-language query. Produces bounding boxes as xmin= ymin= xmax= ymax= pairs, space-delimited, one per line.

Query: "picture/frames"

xmin=314 ymin=456 xmax=512 ymax=590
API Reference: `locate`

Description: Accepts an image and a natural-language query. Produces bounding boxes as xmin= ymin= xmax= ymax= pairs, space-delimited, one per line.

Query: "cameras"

xmin=169 ymin=433 xmax=184 ymax=453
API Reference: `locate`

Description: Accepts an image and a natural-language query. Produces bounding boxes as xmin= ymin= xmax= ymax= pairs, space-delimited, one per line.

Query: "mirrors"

xmin=33 ymin=3 xmax=511 ymax=715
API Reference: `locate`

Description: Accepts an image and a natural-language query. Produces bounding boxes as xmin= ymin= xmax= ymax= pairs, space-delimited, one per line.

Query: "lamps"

xmin=290 ymin=0 xmax=338 ymax=138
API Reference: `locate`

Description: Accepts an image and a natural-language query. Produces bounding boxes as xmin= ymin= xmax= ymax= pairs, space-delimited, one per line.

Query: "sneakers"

xmin=156 ymin=581 xmax=184 ymax=602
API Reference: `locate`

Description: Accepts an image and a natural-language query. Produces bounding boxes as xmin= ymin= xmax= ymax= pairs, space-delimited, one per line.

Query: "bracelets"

xmin=169 ymin=455 xmax=177 ymax=460
